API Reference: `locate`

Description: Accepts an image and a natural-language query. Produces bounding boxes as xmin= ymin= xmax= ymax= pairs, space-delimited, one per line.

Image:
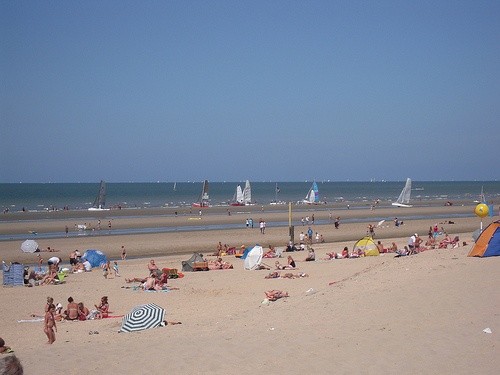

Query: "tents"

xmin=353 ymin=235 xmax=380 ymax=255
xmin=467 ymin=220 xmax=500 ymax=257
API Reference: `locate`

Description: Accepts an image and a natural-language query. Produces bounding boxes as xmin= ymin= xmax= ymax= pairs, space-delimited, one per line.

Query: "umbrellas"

xmin=20 ymin=240 xmax=39 ymax=253
xmin=245 ymin=241 xmax=272 ymax=271
xmin=120 ymin=302 xmax=165 ymax=333
xmin=81 ymin=249 xmax=106 ymax=267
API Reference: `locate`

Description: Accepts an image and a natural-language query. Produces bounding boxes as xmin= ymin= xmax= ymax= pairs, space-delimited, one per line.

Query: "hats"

xmin=415 ymin=233 xmax=419 ymax=237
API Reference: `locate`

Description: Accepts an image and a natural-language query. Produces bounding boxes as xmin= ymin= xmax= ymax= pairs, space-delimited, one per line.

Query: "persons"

xmin=342 ymin=247 xmax=349 ymax=258
xmin=103 ymin=260 xmax=114 ymax=279
xmin=300 ymin=213 xmax=341 ymax=261
xmin=0 ymin=337 xmax=24 ymax=375
xmin=274 ymin=261 xmax=297 ymax=270
xmin=121 ymin=246 xmax=126 ymax=260
xmin=44 ymin=304 xmax=57 ymax=344
xmin=65 ymin=220 xmax=111 ymax=234
xmin=148 ymin=259 xmax=158 ymax=270
xmin=24 ymin=257 xmax=62 ymax=287
xmin=245 ymin=218 xmax=266 ymax=234
xmin=125 ymin=272 xmax=167 ymax=290
xmin=70 ymin=249 xmax=92 ymax=273
xmin=287 ymin=255 xmax=295 ymax=267
xmin=44 ymin=296 xmax=109 ymax=321
xmin=214 ymin=241 xmax=230 ymax=256
xmin=369 ymin=217 xmax=459 ymax=256
xmin=204 ymin=257 xmax=233 ymax=269
xmin=113 ymin=262 xmax=118 ymax=273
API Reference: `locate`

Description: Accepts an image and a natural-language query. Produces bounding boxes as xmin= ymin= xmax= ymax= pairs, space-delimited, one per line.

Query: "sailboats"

xmin=302 ymin=189 xmax=318 ymax=205
xmin=391 ymin=176 xmax=414 ymax=208
xmin=87 ymin=179 xmax=111 ymax=212
xmin=227 ymin=179 xmax=257 ymax=207
xmin=192 ymin=179 xmax=214 ymax=209
